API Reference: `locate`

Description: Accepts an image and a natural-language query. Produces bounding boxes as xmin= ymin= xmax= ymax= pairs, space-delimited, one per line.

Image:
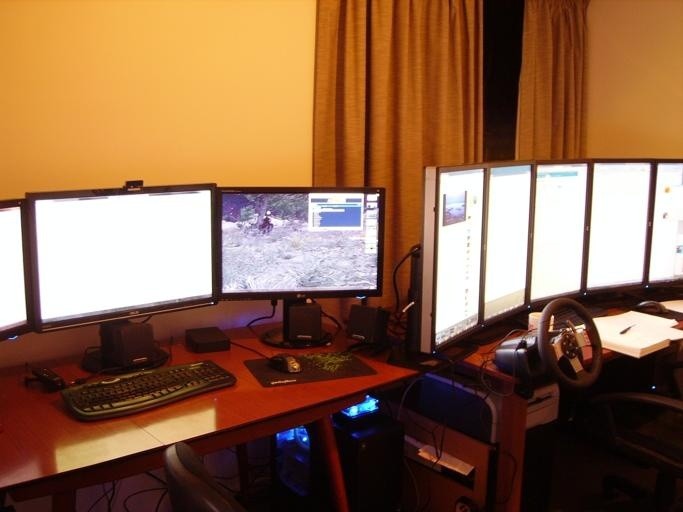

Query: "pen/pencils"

xmin=620 ymin=324 xmax=636 ymax=334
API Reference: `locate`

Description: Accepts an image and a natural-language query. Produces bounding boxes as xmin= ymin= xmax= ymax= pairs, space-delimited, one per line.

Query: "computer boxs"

xmin=394 ymin=369 xmax=561 ymax=512
xmin=236 ymin=395 xmax=406 ymax=512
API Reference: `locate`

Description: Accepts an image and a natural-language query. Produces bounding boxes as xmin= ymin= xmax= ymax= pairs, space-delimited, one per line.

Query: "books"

xmin=583 ymin=313 xmax=671 ymax=361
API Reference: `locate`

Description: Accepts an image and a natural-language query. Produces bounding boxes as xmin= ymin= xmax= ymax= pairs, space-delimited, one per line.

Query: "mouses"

xmin=270 ymin=353 xmax=303 ymax=373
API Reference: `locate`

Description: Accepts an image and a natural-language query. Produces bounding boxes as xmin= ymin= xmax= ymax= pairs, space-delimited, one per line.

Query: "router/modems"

xmin=185 ymin=326 xmax=230 ymax=354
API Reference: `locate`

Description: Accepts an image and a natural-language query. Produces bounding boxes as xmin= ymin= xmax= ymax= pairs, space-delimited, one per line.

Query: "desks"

xmin=0 ymin=320 xmax=422 ymax=511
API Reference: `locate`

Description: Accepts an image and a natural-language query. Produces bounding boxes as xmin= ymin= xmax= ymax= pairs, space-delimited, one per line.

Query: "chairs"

xmin=585 ymin=391 xmax=683 ymax=512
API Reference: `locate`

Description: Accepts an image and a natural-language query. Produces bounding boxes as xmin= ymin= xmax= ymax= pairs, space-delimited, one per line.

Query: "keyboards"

xmin=61 ymin=359 xmax=237 ymax=422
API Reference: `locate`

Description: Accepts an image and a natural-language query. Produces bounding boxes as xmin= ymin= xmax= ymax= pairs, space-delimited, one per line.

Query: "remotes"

xmin=32 ymin=367 xmax=63 ymax=388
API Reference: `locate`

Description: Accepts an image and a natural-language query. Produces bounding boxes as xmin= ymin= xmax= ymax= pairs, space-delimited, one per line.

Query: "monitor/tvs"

xmin=483 ymin=160 xmax=536 ymax=329
xmin=583 ymin=157 xmax=656 ymax=297
xmin=647 ymin=159 xmax=683 ymax=288
xmin=528 ymin=159 xmax=592 ymax=314
xmin=25 ymin=180 xmax=217 ymax=376
xmin=218 ymin=186 xmax=386 ymax=349
xmin=420 ymin=164 xmax=490 ymax=353
xmin=0 ymin=199 xmax=33 ymax=342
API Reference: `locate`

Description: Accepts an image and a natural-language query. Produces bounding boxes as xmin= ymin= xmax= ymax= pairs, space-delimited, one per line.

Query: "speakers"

xmin=287 ymin=304 xmax=321 ymax=340
xmin=346 ymin=305 xmax=396 ymax=356
xmin=111 ymin=323 xmax=154 ymax=367
